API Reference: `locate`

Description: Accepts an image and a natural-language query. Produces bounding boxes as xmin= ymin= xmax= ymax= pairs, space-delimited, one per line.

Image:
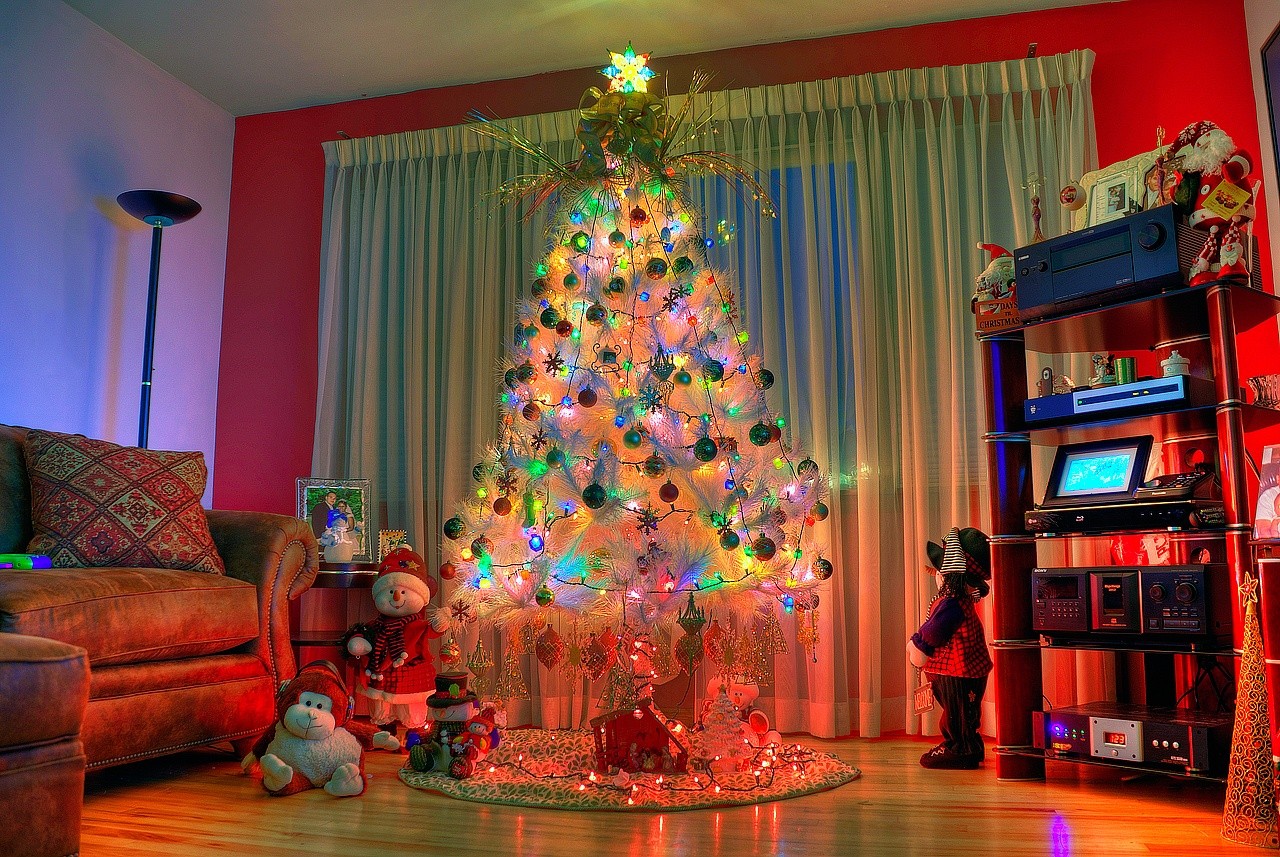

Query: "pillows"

xmin=22 ymin=427 xmax=229 ymax=576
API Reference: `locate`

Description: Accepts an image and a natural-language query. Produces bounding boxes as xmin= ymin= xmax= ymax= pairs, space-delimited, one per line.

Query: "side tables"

xmin=288 ymin=571 xmax=398 ymax=741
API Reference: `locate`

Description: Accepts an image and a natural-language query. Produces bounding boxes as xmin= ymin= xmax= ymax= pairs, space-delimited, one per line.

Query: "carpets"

xmin=396 ymin=727 xmax=863 ymax=812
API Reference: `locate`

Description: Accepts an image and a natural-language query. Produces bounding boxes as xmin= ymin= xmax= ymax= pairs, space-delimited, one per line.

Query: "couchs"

xmin=0 ymin=424 xmax=323 ymax=786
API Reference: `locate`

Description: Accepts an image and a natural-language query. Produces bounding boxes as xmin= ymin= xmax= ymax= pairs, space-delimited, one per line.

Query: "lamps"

xmin=596 ymin=36 xmax=665 ymax=96
xmin=116 ymin=189 xmax=202 ymax=451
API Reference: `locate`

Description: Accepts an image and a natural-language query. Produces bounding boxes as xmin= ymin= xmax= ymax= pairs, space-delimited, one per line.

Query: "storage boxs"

xmin=976 ymin=301 xmax=1023 ymax=337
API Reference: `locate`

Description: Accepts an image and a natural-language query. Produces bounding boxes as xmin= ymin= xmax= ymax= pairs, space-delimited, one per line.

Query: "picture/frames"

xmin=377 ymin=527 xmax=408 ymax=566
xmin=1073 ymin=144 xmax=1179 ymax=232
xmin=295 ymin=476 xmax=379 ymax=566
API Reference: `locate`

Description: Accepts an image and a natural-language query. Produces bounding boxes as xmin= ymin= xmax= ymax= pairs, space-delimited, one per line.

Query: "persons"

xmin=1114 ymin=185 xmax=1125 ymax=212
xmin=325 ymin=498 xmax=361 ymax=554
xmin=1107 ymin=189 xmax=1117 ymax=213
xmin=312 ymin=491 xmax=338 ymax=553
xmin=1143 ymin=164 xmax=1169 ymax=212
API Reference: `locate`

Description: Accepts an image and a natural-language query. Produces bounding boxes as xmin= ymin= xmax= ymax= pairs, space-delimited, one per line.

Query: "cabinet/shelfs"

xmin=990 ymin=283 xmax=1279 ymax=795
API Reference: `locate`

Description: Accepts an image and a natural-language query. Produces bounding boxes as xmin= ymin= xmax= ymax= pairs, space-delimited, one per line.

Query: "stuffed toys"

xmin=899 ymin=529 xmax=994 ymax=772
xmin=1155 ymin=118 xmax=1256 ymax=287
xmin=699 ymin=673 xmax=783 ymax=749
xmin=449 ymin=704 xmax=498 ymax=763
xmin=409 ymin=671 xmax=499 ymax=781
xmin=338 ymin=543 xmax=454 ymax=750
xmin=238 ymin=659 xmax=401 ymax=800
xmin=969 ymin=241 xmax=1020 ymax=316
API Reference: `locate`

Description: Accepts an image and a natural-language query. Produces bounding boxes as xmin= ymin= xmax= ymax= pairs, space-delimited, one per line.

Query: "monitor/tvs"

xmin=1041 ymin=435 xmax=1154 ymax=509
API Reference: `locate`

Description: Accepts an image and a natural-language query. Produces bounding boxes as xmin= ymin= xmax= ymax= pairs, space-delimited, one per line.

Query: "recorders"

xmin=1013 ymin=202 xmax=1263 ymax=324
